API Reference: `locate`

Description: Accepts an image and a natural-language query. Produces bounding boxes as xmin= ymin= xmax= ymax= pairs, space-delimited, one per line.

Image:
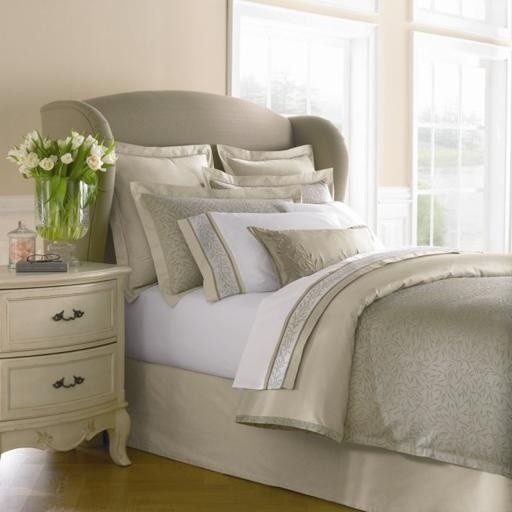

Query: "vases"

xmin=34 ymin=177 xmax=97 ymax=269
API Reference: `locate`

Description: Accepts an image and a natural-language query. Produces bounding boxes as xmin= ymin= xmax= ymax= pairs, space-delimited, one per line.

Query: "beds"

xmin=40 ymin=91 xmax=512 ymax=511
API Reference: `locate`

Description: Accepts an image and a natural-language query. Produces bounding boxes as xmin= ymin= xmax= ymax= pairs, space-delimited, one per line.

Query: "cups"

xmin=9 ymin=221 xmax=35 ymax=270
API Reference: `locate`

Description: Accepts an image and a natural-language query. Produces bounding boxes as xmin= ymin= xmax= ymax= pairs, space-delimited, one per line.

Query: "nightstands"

xmin=0 ymin=262 xmax=132 ymax=467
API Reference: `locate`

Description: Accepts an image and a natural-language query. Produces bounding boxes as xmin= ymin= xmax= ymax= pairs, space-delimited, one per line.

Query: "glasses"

xmin=26 ymin=254 xmax=63 ymax=264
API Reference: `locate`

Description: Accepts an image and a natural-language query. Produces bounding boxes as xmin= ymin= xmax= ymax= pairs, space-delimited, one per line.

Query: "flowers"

xmin=5 ymin=127 xmax=120 ymax=240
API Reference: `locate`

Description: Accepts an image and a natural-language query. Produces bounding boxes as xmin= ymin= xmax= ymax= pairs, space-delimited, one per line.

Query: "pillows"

xmin=129 ymin=181 xmax=302 ymax=309
xmin=201 ymin=166 xmax=334 ymax=204
xmin=216 ymin=144 xmax=315 ymax=177
xmin=177 ymin=211 xmax=383 ymax=303
xmin=110 ymin=141 xmax=215 ymax=303
xmin=279 ymin=200 xmax=374 ymax=229
xmin=247 ymin=225 xmax=377 ymax=288
